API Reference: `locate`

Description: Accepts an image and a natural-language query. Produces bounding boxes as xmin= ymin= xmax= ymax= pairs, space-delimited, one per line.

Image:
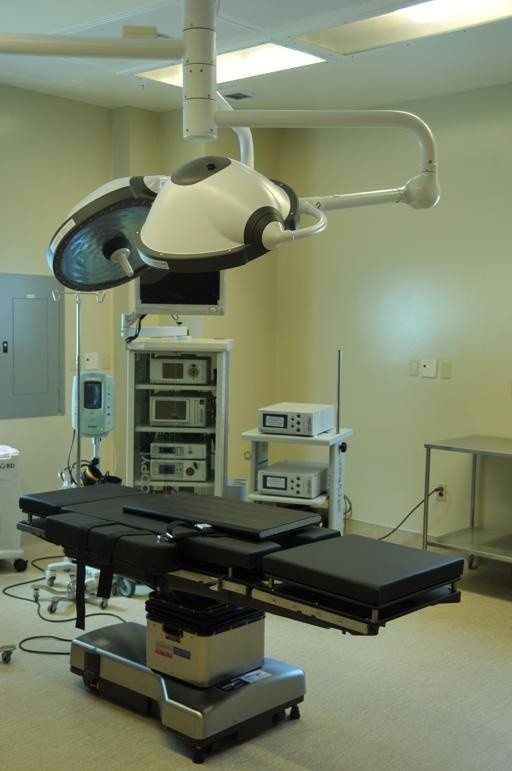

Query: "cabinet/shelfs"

xmin=124 ymin=334 xmax=232 ymax=497
xmin=243 ymin=424 xmax=354 ymax=536
xmin=417 ymin=433 xmax=512 ymax=575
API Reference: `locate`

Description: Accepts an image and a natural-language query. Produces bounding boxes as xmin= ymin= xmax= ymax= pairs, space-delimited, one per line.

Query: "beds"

xmin=16 ymin=480 xmax=461 ymax=764
